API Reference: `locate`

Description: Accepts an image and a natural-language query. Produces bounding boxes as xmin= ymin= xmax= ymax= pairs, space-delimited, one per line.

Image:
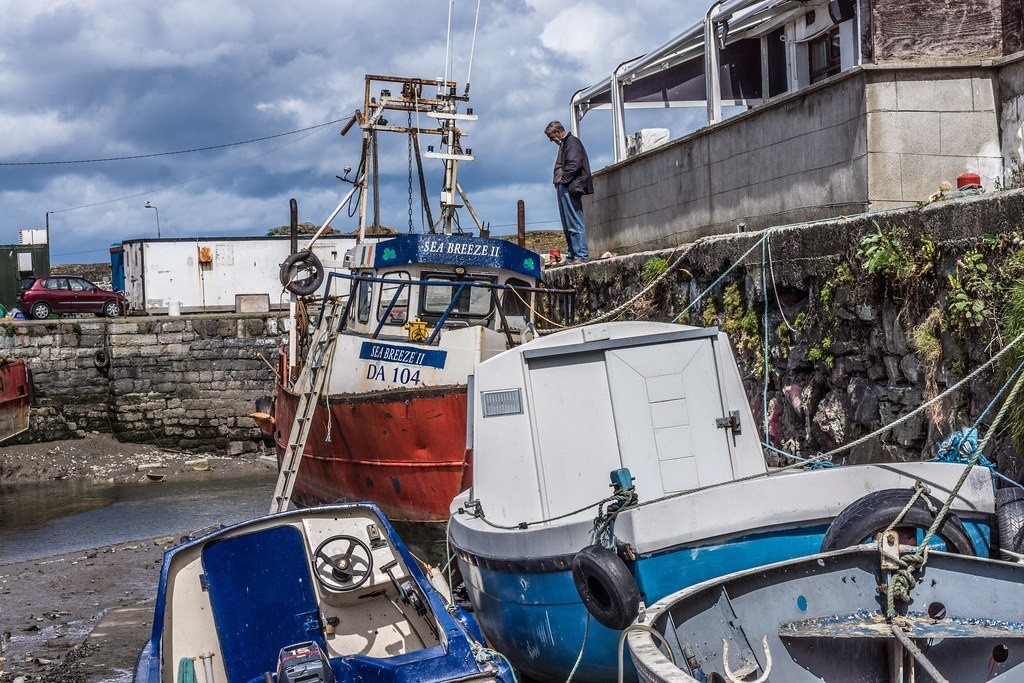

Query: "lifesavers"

xmin=816 ymin=487 xmax=977 ymax=567
xmin=570 ymin=545 xmax=642 ymax=630
xmin=279 ymin=249 xmax=326 ymax=295
xmin=93 ymin=349 xmax=110 ymax=369
xmin=992 ymin=482 xmax=1024 ymax=563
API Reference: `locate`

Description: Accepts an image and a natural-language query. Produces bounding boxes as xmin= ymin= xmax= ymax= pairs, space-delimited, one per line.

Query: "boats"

xmin=625 ymin=527 xmax=1024 ymax=683
xmin=132 ymin=502 xmax=521 ymax=683
xmin=449 ymin=320 xmax=995 ymax=683
xmin=257 ymin=71 xmax=543 ymax=535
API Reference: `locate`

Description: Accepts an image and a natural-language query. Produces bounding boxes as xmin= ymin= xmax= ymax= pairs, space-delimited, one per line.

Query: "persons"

xmin=544 ymin=121 xmax=594 ymax=267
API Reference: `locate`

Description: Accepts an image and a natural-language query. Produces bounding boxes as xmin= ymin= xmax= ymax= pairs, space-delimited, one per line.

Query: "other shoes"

xmin=572 ymin=259 xmax=582 ymax=264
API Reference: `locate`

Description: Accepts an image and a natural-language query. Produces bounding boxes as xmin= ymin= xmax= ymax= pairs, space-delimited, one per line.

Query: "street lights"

xmin=145 ymin=206 xmax=161 ymax=238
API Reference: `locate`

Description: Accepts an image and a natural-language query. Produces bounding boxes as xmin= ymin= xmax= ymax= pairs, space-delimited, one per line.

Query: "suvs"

xmin=16 ymin=275 xmax=128 ymax=319
